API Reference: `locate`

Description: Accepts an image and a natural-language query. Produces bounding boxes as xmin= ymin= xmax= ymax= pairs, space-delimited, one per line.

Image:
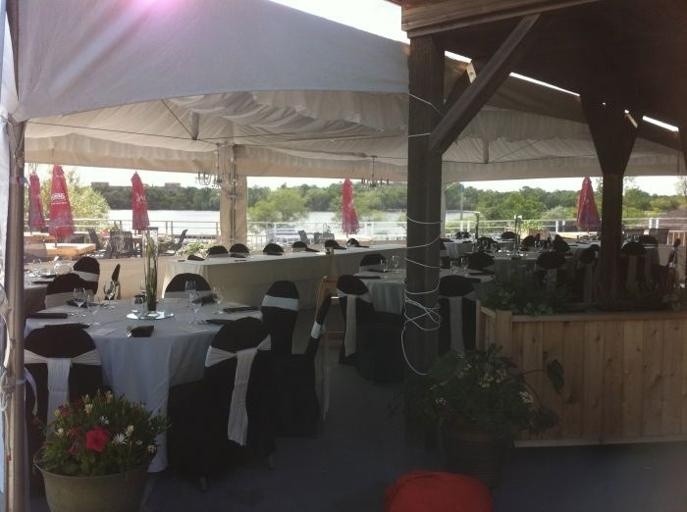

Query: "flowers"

xmin=423 ymin=343 xmax=558 ymax=446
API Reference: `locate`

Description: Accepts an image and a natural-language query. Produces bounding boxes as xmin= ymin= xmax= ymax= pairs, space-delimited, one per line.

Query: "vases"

xmin=440 ymin=418 xmax=502 ymax=472
xmin=32 ymin=445 xmax=156 ymax=512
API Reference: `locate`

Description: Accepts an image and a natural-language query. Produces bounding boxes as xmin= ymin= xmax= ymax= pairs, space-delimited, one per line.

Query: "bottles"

xmin=176 ymin=251 xmax=184 ymax=261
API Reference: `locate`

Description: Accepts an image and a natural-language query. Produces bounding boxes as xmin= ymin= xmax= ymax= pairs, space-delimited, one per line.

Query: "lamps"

xmin=193 ymin=142 xmax=242 ymax=190
xmin=360 ymin=154 xmax=391 ymax=194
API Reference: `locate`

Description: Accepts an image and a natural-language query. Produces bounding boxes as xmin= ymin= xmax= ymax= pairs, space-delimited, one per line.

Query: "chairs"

xmin=332 ymin=228 xmax=686 ymax=382
xmin=19 ymin=228 xmax=332 ymax=482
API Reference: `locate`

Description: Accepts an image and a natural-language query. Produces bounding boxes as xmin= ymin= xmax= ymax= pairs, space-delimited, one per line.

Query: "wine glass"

xmin=380 ymin=255 xmax=470 ymax=278
xmin=71 ymin=279 xmax=202 ymax=327
xmin=212 ymin=285 xmax=224 ymax=315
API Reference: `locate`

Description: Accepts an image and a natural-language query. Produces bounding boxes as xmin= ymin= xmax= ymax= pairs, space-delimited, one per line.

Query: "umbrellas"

xmin=48 ymin=163 xmax=76 ymax=244
xmin=574 ymin=176 xmax=600 ymax=231
xmin=129 ymin=173 xmax=151 ymax=256
xmin=341 ymin=179 xmax=358 ymax=241
xmin=26 ymin=169 xmax=47 ymax=234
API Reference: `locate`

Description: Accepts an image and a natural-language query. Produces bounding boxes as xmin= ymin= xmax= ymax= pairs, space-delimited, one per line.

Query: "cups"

xmin=23 ymin=254 xmax=73 ymax=286
xmin=535 ymin=239 xmax=540 ymax=249
xmin=529 ymin=227 xmax=551 ymax=241
